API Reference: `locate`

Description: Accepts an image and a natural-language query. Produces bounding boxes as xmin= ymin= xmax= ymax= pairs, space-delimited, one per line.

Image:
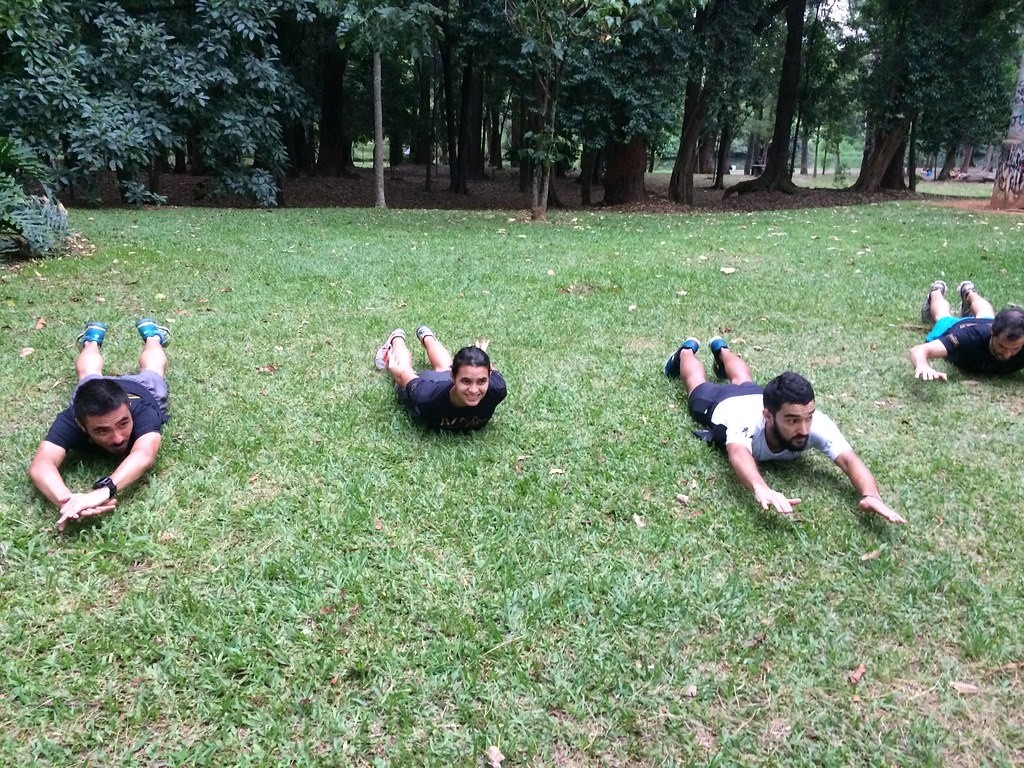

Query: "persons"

xmin=30 ymin=318 xmax=174 ymax=531
xmin=664 ymin=337 xmax=906 ymax=524
xmin=374 ymin=325 xmax=507 ymax=436
xmin=910 ymin=280 xmax=1024 ymax=381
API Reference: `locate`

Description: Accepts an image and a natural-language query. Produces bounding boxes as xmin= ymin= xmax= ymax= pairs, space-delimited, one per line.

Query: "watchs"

xmin=93 ymin=477 xmax=117 ymax=499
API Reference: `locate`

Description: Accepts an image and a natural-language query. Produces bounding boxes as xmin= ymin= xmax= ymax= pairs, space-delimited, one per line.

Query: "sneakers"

xmin=375 ymin=329 xmax=406 ymax=371
xmin=416 ymin=326 xmax=440 ymax=351
xmin=665 ymin=337 xmax=701 ymax=379
xmin=76 ymin=321 xmax=107 ymax=348
xmin=922 ymin=281 xmax=947 ymax=324
xmin=958 ymin=281 xmax=976 ymax=318
xmin=135 ymin=319 xmax=171 ymax=348
xmin=708 ymin=336 xmax=729 ymax=379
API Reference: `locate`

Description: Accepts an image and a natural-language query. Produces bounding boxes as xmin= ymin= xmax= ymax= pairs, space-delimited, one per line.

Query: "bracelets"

xmin=862 ymin=495 xmax=880 ymax=500
